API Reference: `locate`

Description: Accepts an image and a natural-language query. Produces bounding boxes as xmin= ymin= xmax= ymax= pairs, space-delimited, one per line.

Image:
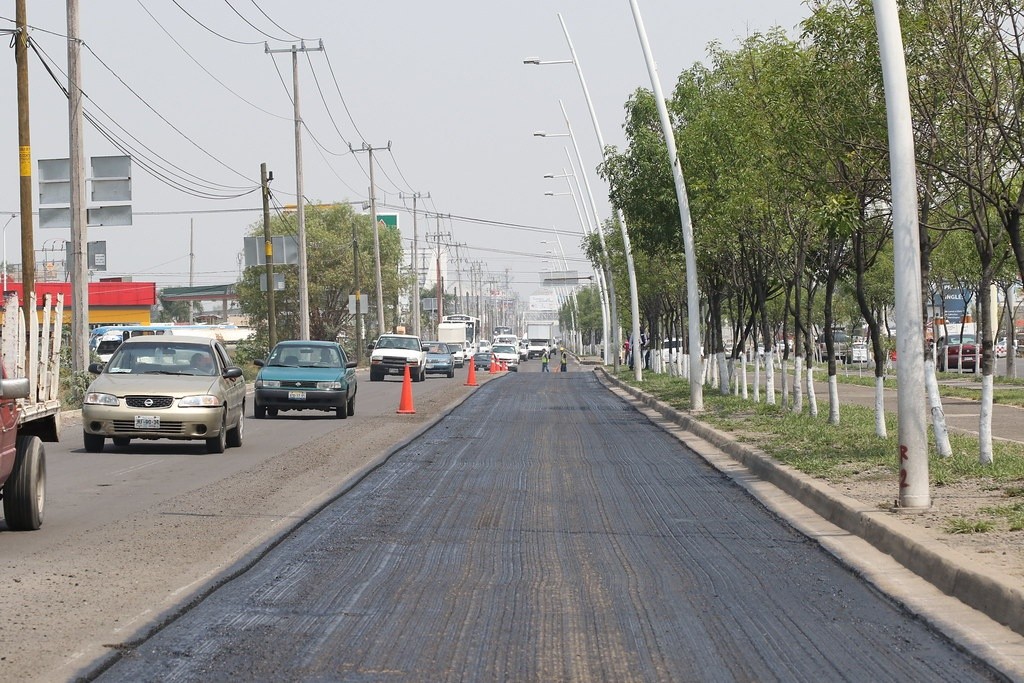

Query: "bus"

xmin=492 ymin=326 xmax=514 ymax=342
xmin=441 ymin=315 xmax=481 ymax=354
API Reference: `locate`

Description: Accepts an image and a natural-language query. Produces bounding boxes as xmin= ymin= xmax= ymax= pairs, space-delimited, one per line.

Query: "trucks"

xmin=526 ymin=320 xmax=554 ymax=358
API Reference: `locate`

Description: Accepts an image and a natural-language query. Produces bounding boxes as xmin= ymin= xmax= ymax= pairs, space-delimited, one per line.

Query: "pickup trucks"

xmin=0 ymin=352 xmax=64 ymax=531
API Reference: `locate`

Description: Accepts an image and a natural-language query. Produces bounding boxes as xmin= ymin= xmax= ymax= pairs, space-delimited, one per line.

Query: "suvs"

xmin=368 ymin=333 xmax=430 ymax=383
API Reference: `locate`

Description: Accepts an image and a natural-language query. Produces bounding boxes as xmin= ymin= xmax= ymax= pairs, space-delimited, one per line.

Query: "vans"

xmin=647 ymin=338 xmax=683 ymax=367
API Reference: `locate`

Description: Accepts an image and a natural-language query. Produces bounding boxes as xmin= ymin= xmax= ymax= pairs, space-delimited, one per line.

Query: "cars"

xmin=471 ymin=352 xmax=498 ymax=370
xmin=419 ymin=340 xmax=456 ymax=378
xmin=700 ymin=339 xmax=794 ymax=361
xmin=82 ymin=335 xmax=247 ymax=454
xmin=813 ymin=327 xmax=853 ymax=365
xmin=851 ymin=342 xmax=869 ymax=362
xmin=437 ymin=334 xmax=563 ymax=373
xmin=253 ymin=340 xmax=359 ymax=420
xmin=929 ymin=334 xmax=984 ymax=374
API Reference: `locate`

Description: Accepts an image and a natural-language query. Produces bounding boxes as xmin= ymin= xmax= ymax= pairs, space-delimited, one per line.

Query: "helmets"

xmin=560 ymin=348 xmax=564 ymax=351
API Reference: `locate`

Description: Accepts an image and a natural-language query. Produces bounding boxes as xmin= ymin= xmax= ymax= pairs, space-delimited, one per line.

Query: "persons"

xmin=559 ymin=348 xmax=567 ymax=372
xmin=541 ymin=349 xmax=550 ymax=372
xmin=190 ymin=353 xmax=206 ymax=370
xmin=642 ymin=329 xmax=651 ymax=370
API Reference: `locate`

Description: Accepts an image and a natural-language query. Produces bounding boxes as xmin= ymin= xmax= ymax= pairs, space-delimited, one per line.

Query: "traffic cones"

xmin=495 ymin=354 xmax=508 ymax=372
xmin=463 ymin=356 xmax=480 ymax=386
xmin=396 ymin=365 xmax=416 ymax=414
xmin=488 ymin=352 xmax=498 ymax=375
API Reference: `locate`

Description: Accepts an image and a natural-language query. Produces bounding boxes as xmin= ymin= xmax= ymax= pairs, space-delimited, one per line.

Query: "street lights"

xmin=522 ymin=11 xmax=643 ymax=383
xmin=533 ymin=102 xmax=619 ymax=376
xmin=534 ymin=145 xmax=614 ymax=368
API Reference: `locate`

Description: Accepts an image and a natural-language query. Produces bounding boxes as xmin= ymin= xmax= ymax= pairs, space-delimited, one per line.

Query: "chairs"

xmin=283 ymin=355 xmax=298 ymax=364
xmin=384 ymin=341 xmax=395 ymax=347
